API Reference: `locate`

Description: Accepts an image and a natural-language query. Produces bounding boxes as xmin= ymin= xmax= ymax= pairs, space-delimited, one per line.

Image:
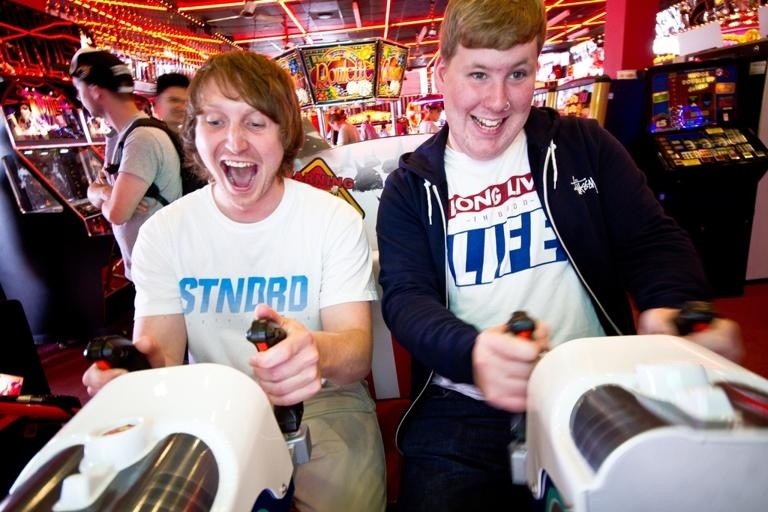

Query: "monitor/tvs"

xmin=1 ymin=103 xmax=93 ymax=151
xmin=86 ymin=110 xmax=113 ymax=141
xmin=11 ymin=151 xmax=76 ymax=211
xmin=644 ymin=58 xmax=741 ymax=136
xmin=81 ymin=145 xmax=105 ymax=183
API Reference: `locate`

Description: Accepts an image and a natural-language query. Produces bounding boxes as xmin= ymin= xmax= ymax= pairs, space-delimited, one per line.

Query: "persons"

xmin=69 ymin=48 xmax=183 ymax=283
xmin=377 ymin=1 xmax=686 ymax=512
xmin=329 ymin=104 xmax=442 ymax=146
xmin=82 ymin=51 xmax=388 ymax=512
xmin=152 ymin=72 xmax=208 ymax=196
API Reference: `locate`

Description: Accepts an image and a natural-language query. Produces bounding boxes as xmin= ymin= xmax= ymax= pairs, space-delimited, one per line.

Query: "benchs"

xmin=360 ymin=243 xmax=422 ymax=511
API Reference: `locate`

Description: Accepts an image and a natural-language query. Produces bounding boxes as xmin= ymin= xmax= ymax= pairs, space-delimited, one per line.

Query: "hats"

xmin=70 ymin=47 xmax=147 ymax=101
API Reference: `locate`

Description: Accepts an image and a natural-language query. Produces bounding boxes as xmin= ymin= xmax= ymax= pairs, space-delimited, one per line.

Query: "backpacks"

xmin=116 ymin=116 xmax=207 ymax=206
